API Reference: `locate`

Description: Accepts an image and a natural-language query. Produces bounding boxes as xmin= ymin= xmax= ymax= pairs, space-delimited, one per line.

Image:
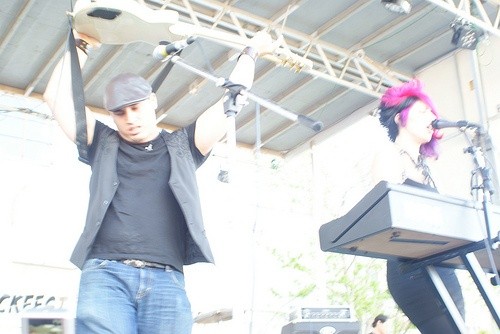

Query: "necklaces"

xmin=145 ymin=142 xmax=154 ymax=150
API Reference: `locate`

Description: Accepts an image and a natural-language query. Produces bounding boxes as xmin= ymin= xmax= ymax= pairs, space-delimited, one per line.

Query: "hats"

xmin=104 ymin=74 xmax=152 ymax=111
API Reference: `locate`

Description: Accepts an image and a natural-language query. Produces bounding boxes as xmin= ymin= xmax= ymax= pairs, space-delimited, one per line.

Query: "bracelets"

xmin=75 ymin=39 xmax=91 ymax=56
xmin=237 ymin=46 xmax=258 ymax=61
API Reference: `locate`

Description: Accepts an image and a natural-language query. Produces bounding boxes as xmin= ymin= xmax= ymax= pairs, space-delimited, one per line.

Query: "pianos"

xmin=319 ymin=180 xmax=499 ymax=268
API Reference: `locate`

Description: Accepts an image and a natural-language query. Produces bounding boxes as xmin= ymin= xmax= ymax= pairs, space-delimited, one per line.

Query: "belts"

xmin=120 ymin=259 xmax=164 ymax=269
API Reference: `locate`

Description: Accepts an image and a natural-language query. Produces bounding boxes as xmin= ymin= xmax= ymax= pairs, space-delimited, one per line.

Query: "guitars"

xmin=73 ymin=0 xmax=313 ymax=75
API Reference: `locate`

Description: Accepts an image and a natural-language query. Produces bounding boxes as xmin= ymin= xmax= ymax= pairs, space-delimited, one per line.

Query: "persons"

xmin=368 ymin=314 xmax=391 ymax=334
xmin=44 ymin=27 xmax=282 ymax=334
xmin=377 ymin=80 xmax=466 ymax=334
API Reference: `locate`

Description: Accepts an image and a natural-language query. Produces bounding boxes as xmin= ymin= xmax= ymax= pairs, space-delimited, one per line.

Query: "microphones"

xmin=432 ymin=119 xmax=476 ymax=129
xmin=153 ymin=36 xmax=197 ymax=60
